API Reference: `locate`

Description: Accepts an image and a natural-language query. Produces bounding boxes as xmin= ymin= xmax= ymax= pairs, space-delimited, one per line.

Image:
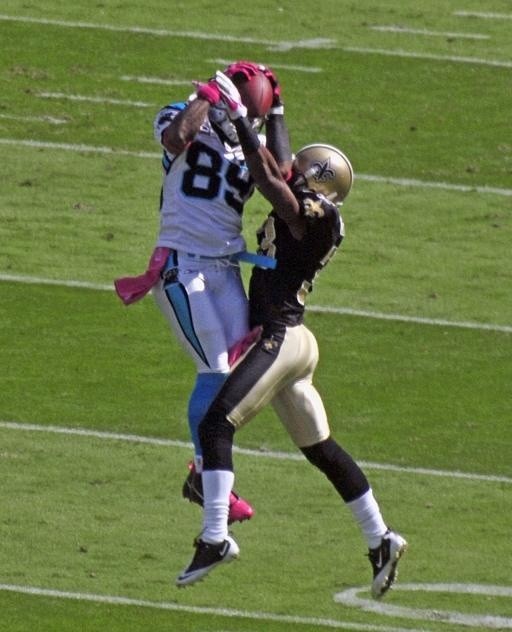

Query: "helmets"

xmin=208 ymin=68 xmax=274 ymax=148
xmin=285 ymin=142 xmax=354 ymax=209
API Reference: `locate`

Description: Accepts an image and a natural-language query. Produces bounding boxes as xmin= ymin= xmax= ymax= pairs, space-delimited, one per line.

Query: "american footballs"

xmin=236 ymin=71 xmax=273 ymax=117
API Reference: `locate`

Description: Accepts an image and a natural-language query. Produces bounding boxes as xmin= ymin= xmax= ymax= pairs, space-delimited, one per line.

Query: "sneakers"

xmin=177 ymin=536 xmax=241 ymax=590
xmin=182 ymin=460 xmax=253 ymax=524
xmin=366 ymin=527 xmax=407 ymax=600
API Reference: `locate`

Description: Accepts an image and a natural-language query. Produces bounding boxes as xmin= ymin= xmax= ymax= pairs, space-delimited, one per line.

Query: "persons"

xmin=176 ymin=70 xmax=407 ymax=600
xmin=151 ymin=61 xmax=292 ymax=523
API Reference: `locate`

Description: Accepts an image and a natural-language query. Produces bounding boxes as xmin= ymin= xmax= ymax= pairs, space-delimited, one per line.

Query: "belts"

xmin=188 ymin=248 xmax=277 ymax=271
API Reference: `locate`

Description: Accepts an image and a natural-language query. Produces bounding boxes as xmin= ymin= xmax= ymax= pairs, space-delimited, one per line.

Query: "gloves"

xmin=192 ymin=59 xmax=285 ymax=121
